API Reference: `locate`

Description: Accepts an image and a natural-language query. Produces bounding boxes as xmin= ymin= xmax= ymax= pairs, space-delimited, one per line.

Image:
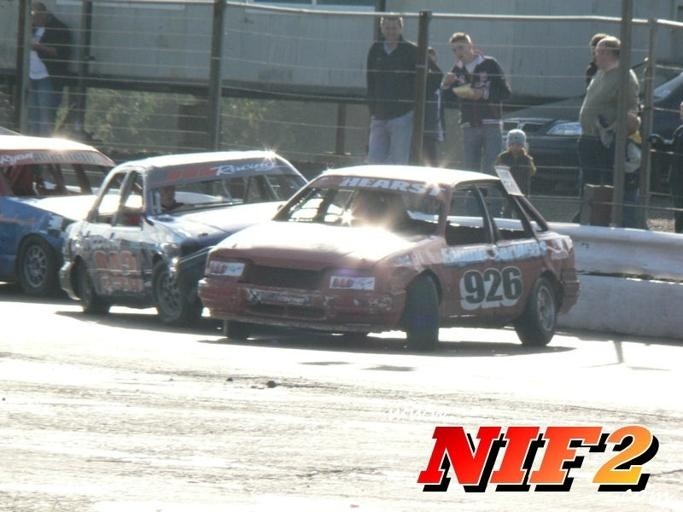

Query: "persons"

xmin=664 ymin=102 xmax=682 ymax=235
xmin=363 ymin=10 xmax=443 ymax=166
xmin=624 ymin=115 xmax=642 ymax=228
xmin=436 ymin=31 xmax=511 ymax=176
xmin=493 ymin=129 xmax=536 ymax=194
xmin=570 ymin=32 xmax=610 ymax=224
xmin=578 ymin=37 xmax=640 ymax=138
xmin=159 ymin=185 xmax=184 ymax=213
xmin=25 ymin=1 xmax=73 ymax=138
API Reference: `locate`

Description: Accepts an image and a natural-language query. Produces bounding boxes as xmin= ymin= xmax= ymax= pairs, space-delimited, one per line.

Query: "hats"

xmin=506 ymin=127 xmax=526 ymax=147
xmin=613 ymin=111 xmax=642 ymax=138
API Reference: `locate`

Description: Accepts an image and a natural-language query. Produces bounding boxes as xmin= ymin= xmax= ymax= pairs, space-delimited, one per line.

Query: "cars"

xmin=0 ymin=129 xmax=145 ymax=297
xmin=494 ymin=52 xmax=683 ymax=198
xmin=195 ymin=162 xmax=581 ymax=352
xmin=56 ymin=145 xmax=348 ymax=329
